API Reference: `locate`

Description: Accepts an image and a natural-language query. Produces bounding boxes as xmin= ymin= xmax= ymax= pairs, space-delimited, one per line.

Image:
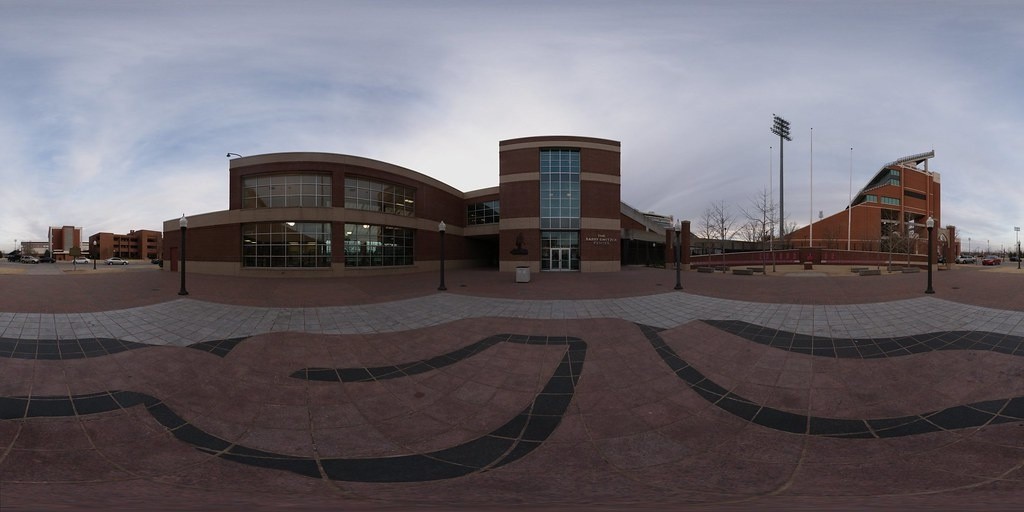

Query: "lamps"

xmin=227 ymin=153 xmax=243 ymax=158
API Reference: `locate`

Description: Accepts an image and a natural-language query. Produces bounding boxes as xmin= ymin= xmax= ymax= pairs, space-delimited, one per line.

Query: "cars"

xmin=954 ymin=255 xmax=977 ymax=264
xmin=982 ymin=255 xmax=1002 ymax=266
xmin=105 ymin=257 xmax=129 ymax=266
xmin=938 ymin=256 xmax=947 ymax=264
xmin=20 ymin=256 xmax=39 ymax=264
xmin=1010 ymin=254 xmax=1020 ymax=262
xmin=72 ymin=256 xmax=91 ymax=264
xmin=39 ymin=256 xmax=56 ymax=263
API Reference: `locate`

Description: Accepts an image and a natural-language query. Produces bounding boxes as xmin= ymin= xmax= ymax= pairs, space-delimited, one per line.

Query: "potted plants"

xmin=516 ymin=265 xmax=530 ymax=282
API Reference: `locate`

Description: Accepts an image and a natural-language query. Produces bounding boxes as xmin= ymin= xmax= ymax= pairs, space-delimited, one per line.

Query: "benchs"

xmin=698 ymin=267 xmax=714 ymax=273
xmin=851 ymin=268 xmax=868 ymax=273
xmin=747 ymin=268 xmax=764 ymax=272
xmin=733 ymin=270 xmax=753 ymax=275
xmin=903 ymin=268 xmax=920 ymax=272
xmin=887 ymin=266 xmax=903 ymax=271
xmin=715 ymin=266 xmax=728 ymax=270
xmin=859 ymin=270 xmax=881 ymax=275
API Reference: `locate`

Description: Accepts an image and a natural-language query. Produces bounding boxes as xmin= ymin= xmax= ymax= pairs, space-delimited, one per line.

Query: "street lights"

xmin=769 ymin=114 xmax=792 ymax=250
xmin=1017 ymin=240 xmax=1021 ymax=269
